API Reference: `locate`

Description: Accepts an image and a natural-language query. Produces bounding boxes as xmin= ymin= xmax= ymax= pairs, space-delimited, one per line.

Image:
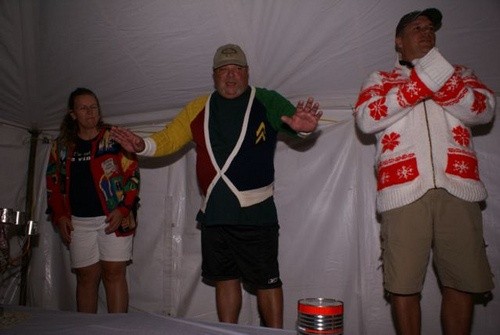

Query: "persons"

xmin=45 ymin=85 xmax=141 ymax=314
xmin=111 ymin=41 xmax=323 ymax=330
xmin=353 ymin=5 xmax=496 ymax=335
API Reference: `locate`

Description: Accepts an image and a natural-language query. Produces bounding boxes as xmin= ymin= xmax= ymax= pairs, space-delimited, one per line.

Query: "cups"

xmin=296 ymin=298 xmax=344 ymax=335
xmin=0 ymin=208 xmax=39 ymax=236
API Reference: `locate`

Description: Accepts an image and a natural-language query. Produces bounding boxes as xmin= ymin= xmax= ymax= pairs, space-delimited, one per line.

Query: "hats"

xmin=394 ymin=6 xmax=443 ymax=38
xmin=213 ymin=43 xmax=247 ymax=69
